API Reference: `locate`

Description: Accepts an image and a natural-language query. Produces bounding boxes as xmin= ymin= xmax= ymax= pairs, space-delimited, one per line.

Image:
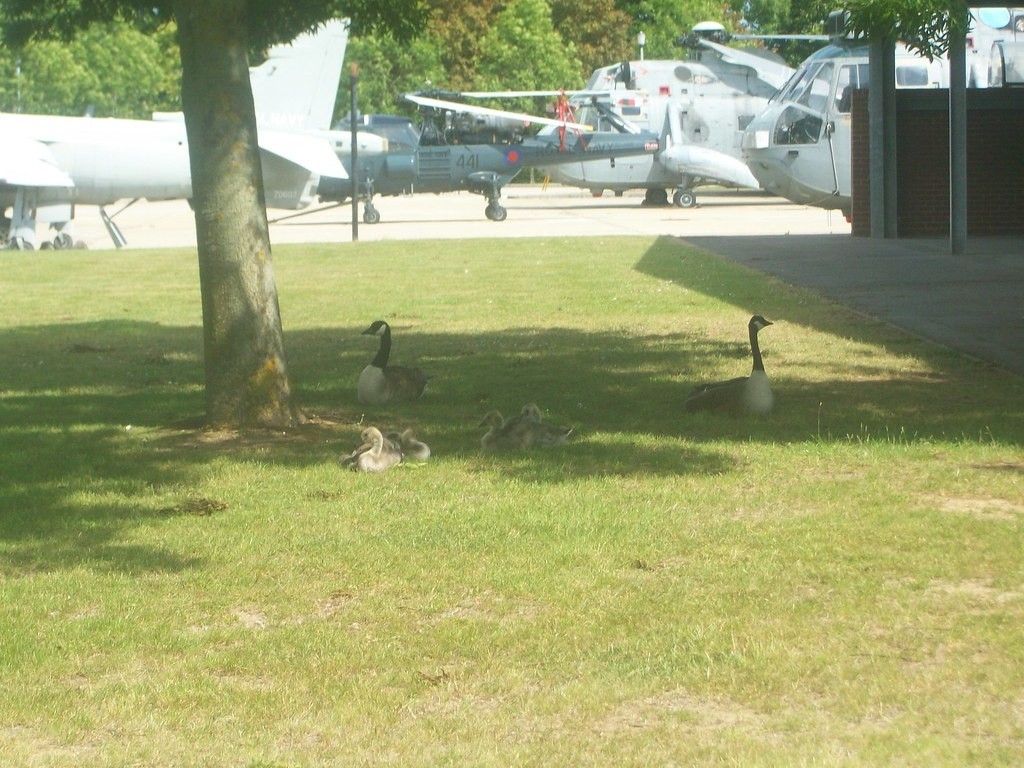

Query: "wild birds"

xmin=338 ymin=426 xmax=430 ymax=474
xmin=357 ymin=321 xmax=433 ymax=409
xmin=478 ymin=403 xmax=573 ymax=450
xmin=684 ymin=316 xmax=774 ymax=416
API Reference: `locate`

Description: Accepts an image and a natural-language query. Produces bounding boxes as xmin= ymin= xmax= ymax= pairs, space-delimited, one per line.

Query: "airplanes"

xmin=0 ymin=13 xmax=356 ymax=255
xmin=316 ymin=9 xmax=1024 ymax=227
